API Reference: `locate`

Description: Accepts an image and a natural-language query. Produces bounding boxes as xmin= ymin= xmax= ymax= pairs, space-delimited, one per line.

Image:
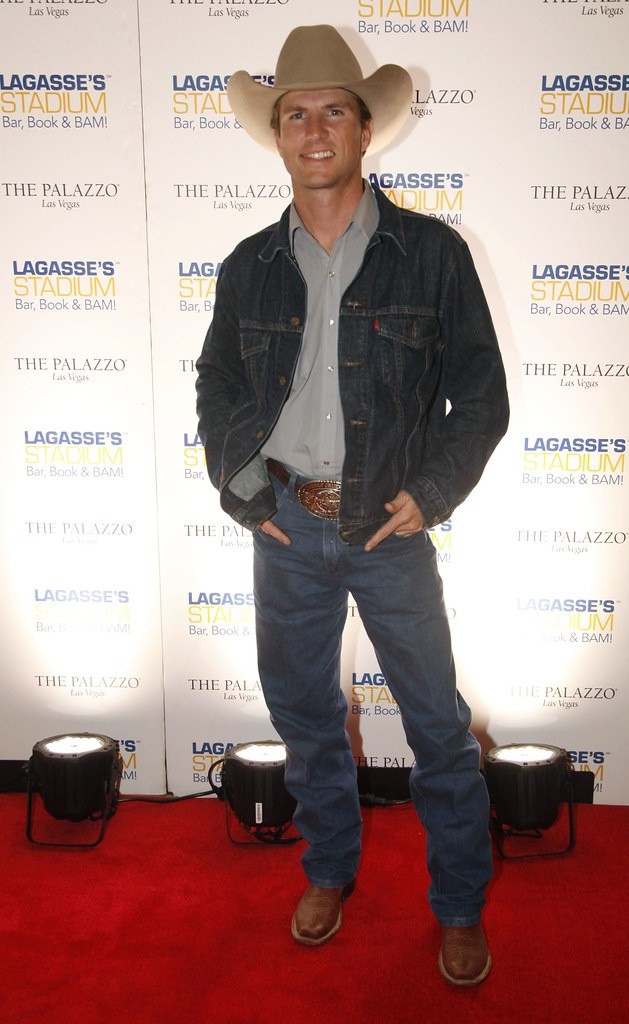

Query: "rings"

xmin=395 ymin=532 xmax=399 ymax=535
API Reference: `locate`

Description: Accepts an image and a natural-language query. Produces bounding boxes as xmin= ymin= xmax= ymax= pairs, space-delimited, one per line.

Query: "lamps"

xmin=480 ymin=743 xmax=577 ymax=860
xmin=24 ymin=732 xmax=125 ymax=847
xmin=206 ymin=740 xmax=303 ymax=848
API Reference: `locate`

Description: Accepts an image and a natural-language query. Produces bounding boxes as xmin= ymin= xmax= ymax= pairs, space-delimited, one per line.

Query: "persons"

xmin=194 ymin=25 xmax=510 ymax=985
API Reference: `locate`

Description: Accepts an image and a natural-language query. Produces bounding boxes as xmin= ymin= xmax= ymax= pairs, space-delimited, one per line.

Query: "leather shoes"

xmin=438 ymin=923 xmax=491 ymax=985
xmin=291 ymin=885 xmax=344 ymax=945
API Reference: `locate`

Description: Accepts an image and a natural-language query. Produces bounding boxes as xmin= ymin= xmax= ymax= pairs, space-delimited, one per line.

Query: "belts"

xmin=265 ymin=458 xmax=342 ymax=521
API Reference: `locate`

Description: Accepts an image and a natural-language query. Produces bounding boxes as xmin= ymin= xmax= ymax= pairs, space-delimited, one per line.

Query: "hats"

xmin=226 ymin=24 xmax=413 ymax=160
